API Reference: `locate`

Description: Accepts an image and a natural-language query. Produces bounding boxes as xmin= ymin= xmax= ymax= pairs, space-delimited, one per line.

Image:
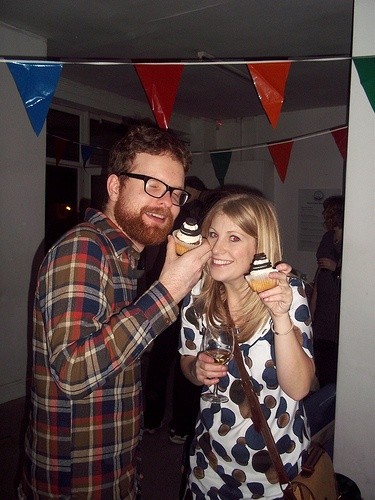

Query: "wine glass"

xmin=200 ymin=325 xmax=234 ymax=403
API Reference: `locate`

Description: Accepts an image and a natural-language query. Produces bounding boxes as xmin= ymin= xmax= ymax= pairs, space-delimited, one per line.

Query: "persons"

xmin=178 ymin=194 xmax=315 ymax=500
xmin=308 ymin=195 xmax=345 ymax=388
xmin=136 ymin=177 xmax=207 ymax=442
xmin=18 ymin=125 xmax=213 ymax=500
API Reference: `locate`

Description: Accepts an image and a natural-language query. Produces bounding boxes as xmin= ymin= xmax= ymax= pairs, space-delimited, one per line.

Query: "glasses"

xmin=118 ymin=171 xmax=191 ymax=206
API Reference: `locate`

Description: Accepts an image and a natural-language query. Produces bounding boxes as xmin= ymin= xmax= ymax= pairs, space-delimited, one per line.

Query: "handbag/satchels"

xmin=284 ymin=446 xmax=338 ymax=500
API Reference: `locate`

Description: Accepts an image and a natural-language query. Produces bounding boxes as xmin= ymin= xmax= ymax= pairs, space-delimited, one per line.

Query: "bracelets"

xmin=271 ymin=319 xmax=293 ymax=335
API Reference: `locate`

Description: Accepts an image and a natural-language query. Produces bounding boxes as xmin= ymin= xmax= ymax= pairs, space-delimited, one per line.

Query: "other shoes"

xmin=168 ymin=427 xmax=189 ymax=445
xmin=144 ymin=419 xmax=167 ymax=434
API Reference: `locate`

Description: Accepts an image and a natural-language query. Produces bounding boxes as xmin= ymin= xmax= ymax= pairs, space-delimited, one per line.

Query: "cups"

xmin=318 ymin=256 xmax=329 ymax=272
